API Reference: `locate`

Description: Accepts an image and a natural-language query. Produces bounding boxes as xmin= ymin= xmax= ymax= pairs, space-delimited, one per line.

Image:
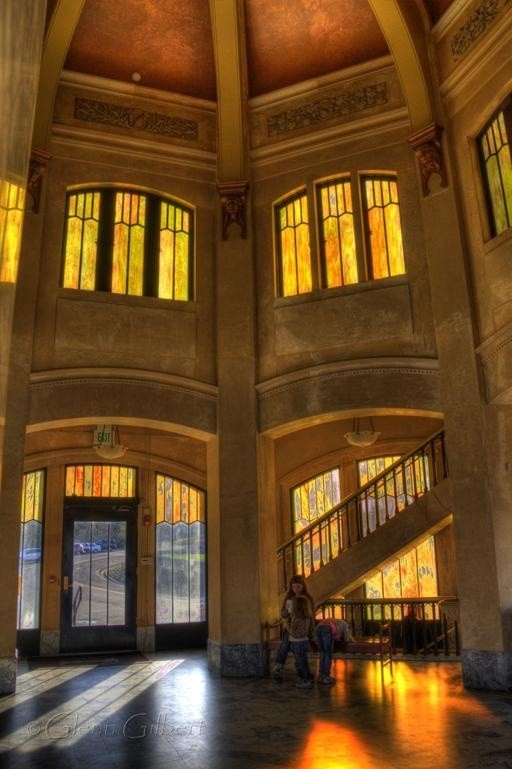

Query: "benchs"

xmin=264 ymin=617 xmax=394 ymax=682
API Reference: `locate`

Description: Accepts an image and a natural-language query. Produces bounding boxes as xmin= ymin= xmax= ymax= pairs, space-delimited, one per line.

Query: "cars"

xmin=19 ymin=537 xmax=118 ymax=566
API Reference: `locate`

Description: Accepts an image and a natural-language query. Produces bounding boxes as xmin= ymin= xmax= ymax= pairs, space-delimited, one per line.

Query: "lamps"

xmin=345 ymin=417 xmax=381 ymax=449
xmin=93 ymin=422 xmax=128 ymax=461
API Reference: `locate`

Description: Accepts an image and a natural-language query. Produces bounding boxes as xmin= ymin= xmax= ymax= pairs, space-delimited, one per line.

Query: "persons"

xmin=271 ymin=575 xmax=321 ymax=674
xmin=282 ymin=594 xmax=317 ymax=689
xmin=313 ymin=617 xmax=357 ymax=685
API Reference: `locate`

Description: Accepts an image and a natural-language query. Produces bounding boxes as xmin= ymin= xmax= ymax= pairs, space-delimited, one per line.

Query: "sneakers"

xmin=269 ymin=663 xmax=335 ymax=689
